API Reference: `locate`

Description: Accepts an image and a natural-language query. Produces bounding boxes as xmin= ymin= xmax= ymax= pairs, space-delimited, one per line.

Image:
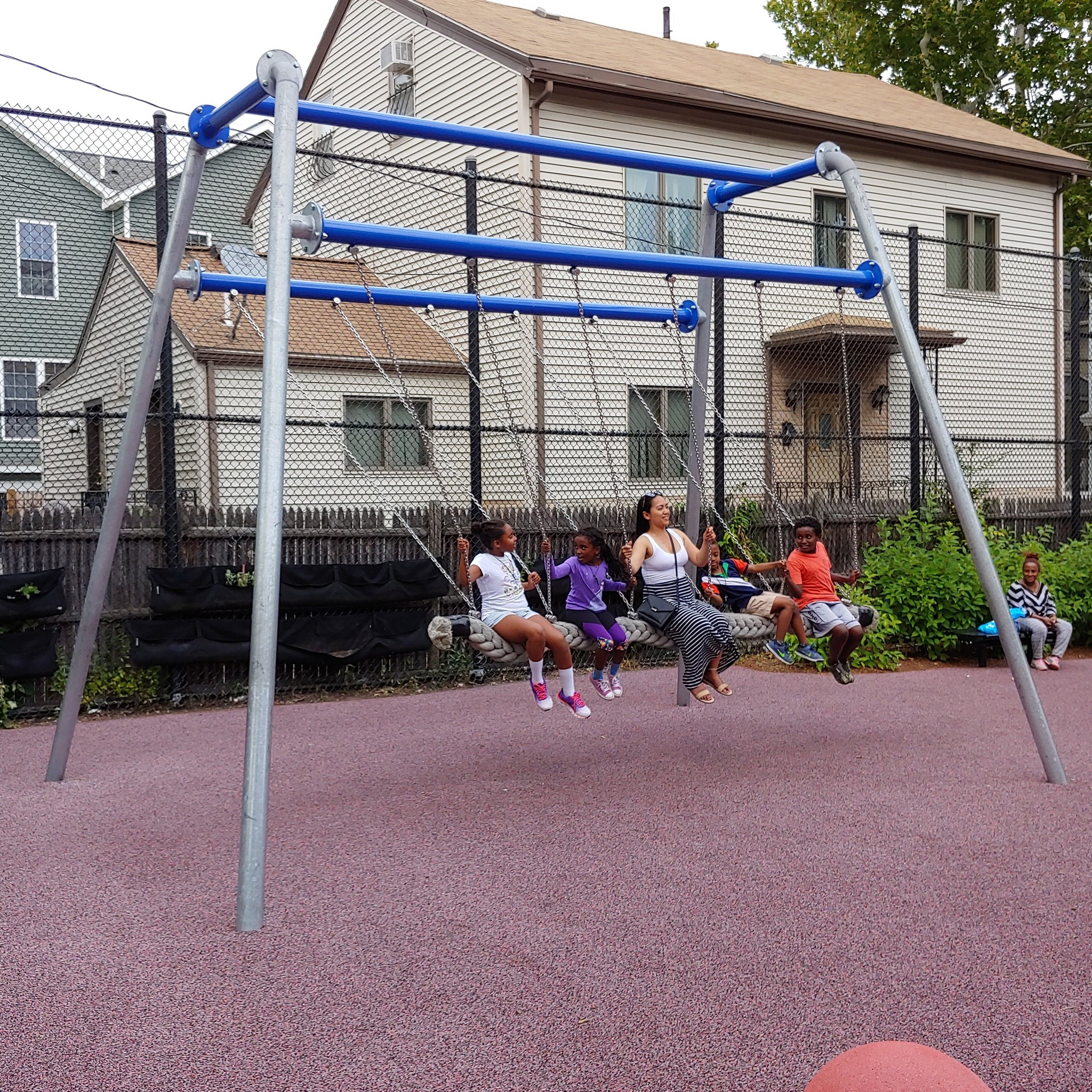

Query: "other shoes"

xmin=826 ymin=656 xmax=851 ymax=684
xmin=839 ymin=658 xmax=854 ymax=683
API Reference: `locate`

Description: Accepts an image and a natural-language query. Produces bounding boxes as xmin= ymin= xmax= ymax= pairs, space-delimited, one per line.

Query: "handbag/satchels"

xmin=976 ymin=607 xmax=1028 ymax=634
xmin=634 ymin=530 xmax=680 ymax=632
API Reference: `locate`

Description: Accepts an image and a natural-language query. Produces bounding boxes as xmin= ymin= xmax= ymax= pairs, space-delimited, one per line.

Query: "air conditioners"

xmin=378 ymin=39 xmax=413 ymax=74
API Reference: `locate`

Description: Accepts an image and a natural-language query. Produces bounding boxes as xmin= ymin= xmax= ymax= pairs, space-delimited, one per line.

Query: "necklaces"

xmin=712 ymin=565 xmax=728 ymax=601
xmin=1024 ymin=581 xmax=1036 ymax=595
xmin=488 ymin=552 xmax=517 ymax=580
xmin=586 ymin=560 xmax=599 ymax=586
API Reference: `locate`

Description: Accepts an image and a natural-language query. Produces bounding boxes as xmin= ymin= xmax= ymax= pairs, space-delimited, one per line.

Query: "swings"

xmin=231 ymin=245 xmax=880 ymax=666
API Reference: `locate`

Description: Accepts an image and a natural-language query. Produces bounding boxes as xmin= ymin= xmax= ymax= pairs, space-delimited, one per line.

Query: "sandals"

xmin=690 ymin=682 xmax=714 ymax=704
xmin=702 ymin=668 xmax=732 ymax=696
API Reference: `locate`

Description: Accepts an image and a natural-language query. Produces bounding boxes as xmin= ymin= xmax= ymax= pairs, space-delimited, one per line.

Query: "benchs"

xmin=963 ymin=624 xmax=1055 ymax=662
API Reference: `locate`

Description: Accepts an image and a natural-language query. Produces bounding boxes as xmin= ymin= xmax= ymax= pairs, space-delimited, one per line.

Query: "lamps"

xmin=782 ymin=381 xmax=806 ymax=414
xmin=869 ymin=383 xmax=895 ymax=416
xmin=780 ymin=419 xmax=798 ymax=447
xmin=67 ymin=421 xmax=83 ymax=441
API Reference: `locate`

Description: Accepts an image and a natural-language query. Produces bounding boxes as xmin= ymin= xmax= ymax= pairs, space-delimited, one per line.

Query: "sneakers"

xmin=589 ymin=672 xmax=614 ymax=700
xmin=794 ymin=642 xmax=824 ymax=662
xmin=1030 ymin=658 xmax=1048 ymax=671
xmin=765 ymin=639 xmax=794 ymax=665
xmin=1044 ymin=656 xmax=1060 ymax=669
xmin=556 ymin=687 xmax=592 ymax=719
xmin=530 ymin=674 xmax=553 ymax=712
xmin=606 ymin=666 xmax=623 ymax=697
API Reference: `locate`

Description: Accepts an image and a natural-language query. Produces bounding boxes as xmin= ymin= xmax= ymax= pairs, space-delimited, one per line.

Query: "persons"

xmin=457 ymin=519 xmax=591 ymax=719
xmin=1006 ymin=552 xmax=1072 ymax=671
xmin=619 ymin=491 xmax=742 ymax=704
xmin=696 ymin=536 xmax=824 ymax=666
xmin=779 ymin=517 xmax=864 ymax=685
xmin=541 ymin=526 xmax=637 ymax=701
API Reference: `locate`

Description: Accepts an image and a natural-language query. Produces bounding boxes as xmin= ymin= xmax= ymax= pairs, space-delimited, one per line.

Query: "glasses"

xmin=642 ymin=491 xmax=663 ymax=509
